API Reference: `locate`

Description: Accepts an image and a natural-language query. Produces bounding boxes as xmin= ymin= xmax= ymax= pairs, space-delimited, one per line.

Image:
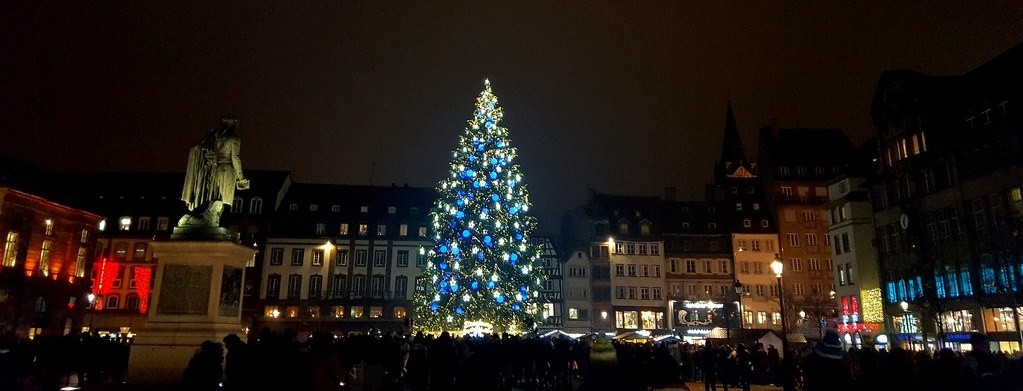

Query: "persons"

xmin=612 ymin=331 xmax=1023 ymax=391
xmin=0 ymin=329 xmax=130 ymax=391
xmin=181 ymin=114 xmax=250 ymax=227
xmin=182 ymin=327 xmax=591 ymax=391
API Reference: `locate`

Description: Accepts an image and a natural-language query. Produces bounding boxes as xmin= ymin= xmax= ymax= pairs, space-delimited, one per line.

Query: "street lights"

xmin=900 ymin=300 xmax=912 ymax=350
xmin=770 ymin=252 xmax=792 ymax=391
xmin=734 ymin=279 xmax=744 ymax=342
